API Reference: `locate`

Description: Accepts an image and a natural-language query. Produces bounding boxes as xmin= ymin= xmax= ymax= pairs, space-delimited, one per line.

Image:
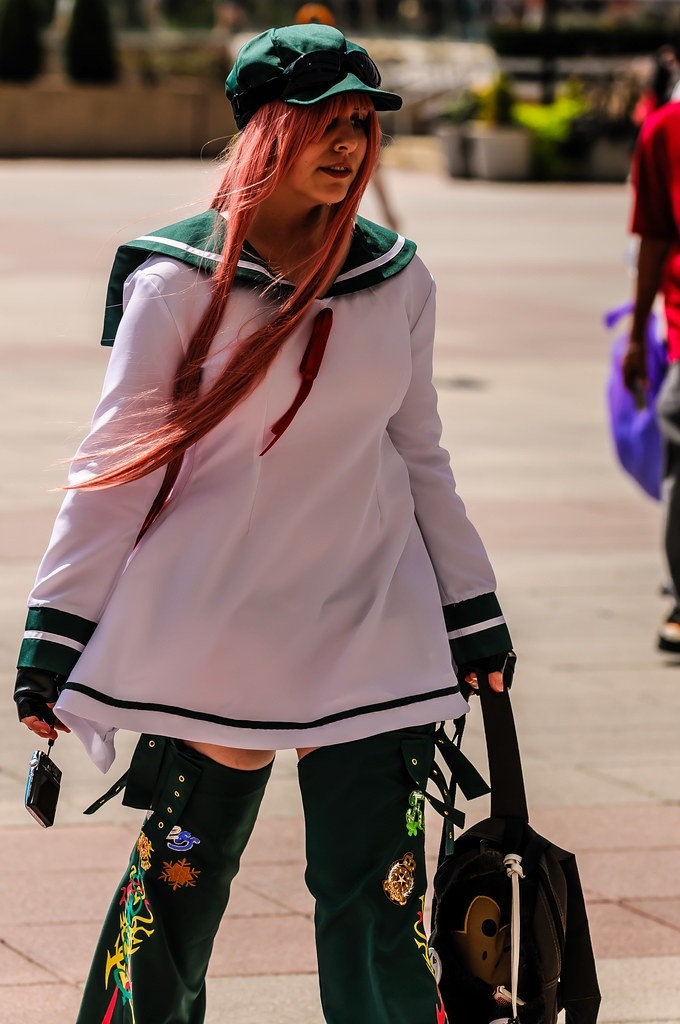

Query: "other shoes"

xmin=656 ymin=603 xmax=679 ymax=665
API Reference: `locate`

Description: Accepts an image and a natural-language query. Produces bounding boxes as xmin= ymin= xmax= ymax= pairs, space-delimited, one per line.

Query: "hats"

xmin=223 ymin=21 xmax=403 ymax=129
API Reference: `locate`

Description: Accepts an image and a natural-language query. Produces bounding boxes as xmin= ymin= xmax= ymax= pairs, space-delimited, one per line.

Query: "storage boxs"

xmin=434 ymin=122 xmax=534 ymax=179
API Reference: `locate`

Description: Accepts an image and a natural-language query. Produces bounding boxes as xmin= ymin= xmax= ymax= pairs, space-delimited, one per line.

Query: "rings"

xmin=28 ymin=727 xmax=32 ymax=731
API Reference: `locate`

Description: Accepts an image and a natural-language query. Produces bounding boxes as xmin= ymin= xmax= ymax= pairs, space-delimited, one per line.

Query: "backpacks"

xmin=422 ymin=673 xmax=604 ymax=1024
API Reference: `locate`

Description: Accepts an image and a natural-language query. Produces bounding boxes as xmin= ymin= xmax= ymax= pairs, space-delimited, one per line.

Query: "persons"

xmin=617 ymin=46 xmax=680 ymax=652
xmin=13 ymin=23 xmax=603 ymax=1024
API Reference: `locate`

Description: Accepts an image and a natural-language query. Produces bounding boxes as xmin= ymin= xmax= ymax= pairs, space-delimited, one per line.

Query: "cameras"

xmin=24 ymin=751 xmax=63 ymax=828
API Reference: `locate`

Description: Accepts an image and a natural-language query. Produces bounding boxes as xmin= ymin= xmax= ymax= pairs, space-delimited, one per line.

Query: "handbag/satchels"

xmin=602 ymin=300 xmax=667 ymax=502
